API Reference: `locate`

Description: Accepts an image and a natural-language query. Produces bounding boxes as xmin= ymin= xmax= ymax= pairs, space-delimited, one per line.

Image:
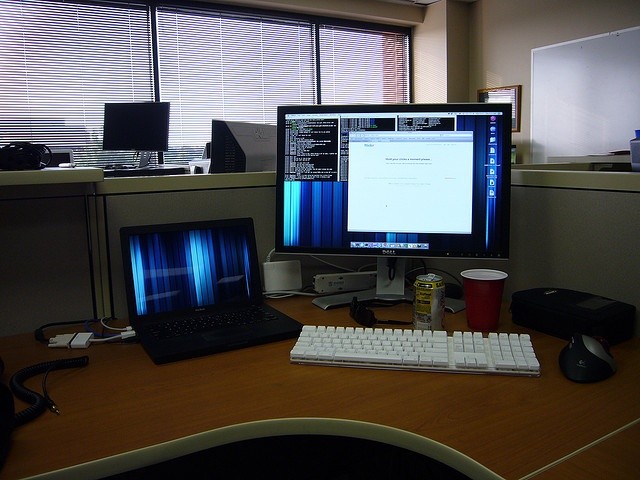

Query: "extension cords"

xmin=314 ymin=272 xmax=377 ymax=293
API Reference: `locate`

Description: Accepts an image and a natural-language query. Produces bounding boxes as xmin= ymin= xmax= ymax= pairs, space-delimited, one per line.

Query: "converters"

xmin=263 ymin=260 xmax=303 ymax=291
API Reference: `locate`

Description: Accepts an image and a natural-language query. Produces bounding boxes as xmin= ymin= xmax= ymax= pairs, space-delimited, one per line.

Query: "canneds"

xmin=412 ymin=273 xmax=446 ymax=334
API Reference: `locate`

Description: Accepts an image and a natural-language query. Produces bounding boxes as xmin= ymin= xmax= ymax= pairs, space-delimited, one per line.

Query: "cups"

xmin=460 ymin=269 xmax=509 ymax=332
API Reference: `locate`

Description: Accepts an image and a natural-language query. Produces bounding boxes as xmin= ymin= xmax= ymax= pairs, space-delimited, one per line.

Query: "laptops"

xmin=118 ymin=217 xmax=304 ymax=365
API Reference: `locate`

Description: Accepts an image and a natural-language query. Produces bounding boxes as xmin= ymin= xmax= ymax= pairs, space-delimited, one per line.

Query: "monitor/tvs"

xmin=276 ymin=103 xmax=511 ymax=312
xmin=210 ymin=119 xmax=276 ymax=173
xmin=104 ymin=101 xmax=168 ymax=168
xmin=42 ymin=148 xmax=74 ymax=167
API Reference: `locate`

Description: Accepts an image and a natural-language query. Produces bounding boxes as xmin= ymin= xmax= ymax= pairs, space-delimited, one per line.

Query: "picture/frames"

xmin=476 ymin=84 xmax=521 ymax=132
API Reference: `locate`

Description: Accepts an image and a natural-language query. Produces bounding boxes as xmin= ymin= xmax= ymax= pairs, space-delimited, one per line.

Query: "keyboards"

xmin=289 ymin=324 xmax=540 ymax=378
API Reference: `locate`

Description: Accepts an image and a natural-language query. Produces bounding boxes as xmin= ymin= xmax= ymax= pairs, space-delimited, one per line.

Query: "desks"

xmin=0 ymin=277 xmax=640 ymax=480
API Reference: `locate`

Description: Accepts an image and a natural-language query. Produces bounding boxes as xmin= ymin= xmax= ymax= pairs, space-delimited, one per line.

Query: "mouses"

xmin=558 ymin=330 xmax=619 ymax=384
